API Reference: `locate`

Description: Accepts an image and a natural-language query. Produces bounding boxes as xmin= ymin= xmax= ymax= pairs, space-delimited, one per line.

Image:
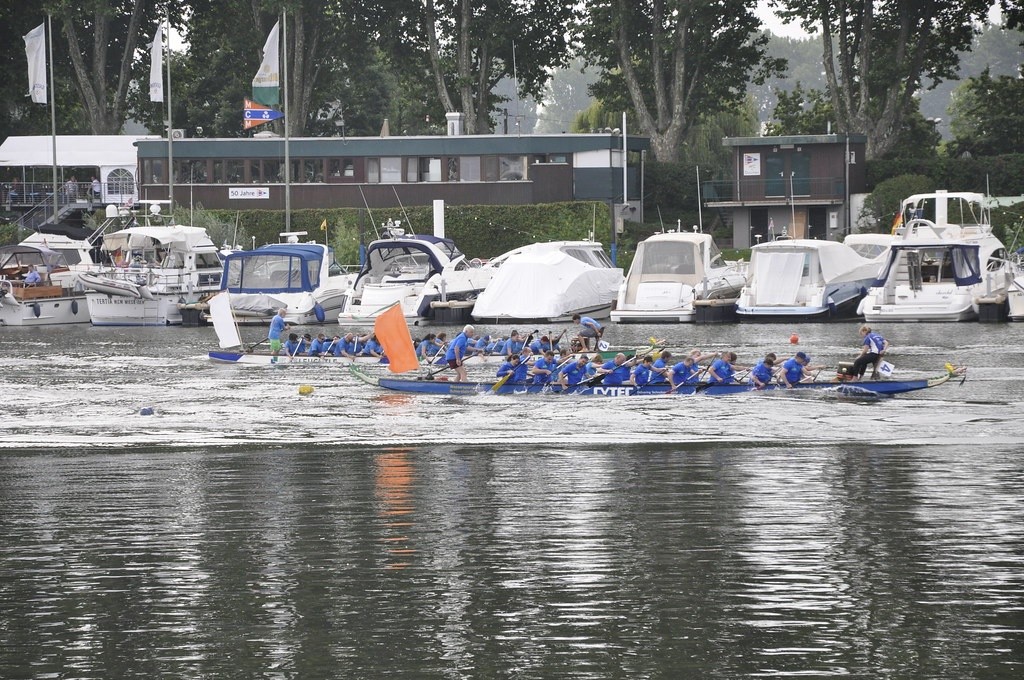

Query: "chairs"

xmin=678 ymin=263 xmax=696 ymax=274
xmin=649 ymin=264 xmax=671 ymax=274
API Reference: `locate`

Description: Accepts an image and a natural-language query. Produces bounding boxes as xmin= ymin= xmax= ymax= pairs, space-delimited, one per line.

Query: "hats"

xmin=797 ymin=352 xmax=807 ymax=360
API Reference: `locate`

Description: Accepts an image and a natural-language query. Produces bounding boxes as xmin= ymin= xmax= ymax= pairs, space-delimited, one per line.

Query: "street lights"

xmin=926 ymin=116 xmax=942 ymax=153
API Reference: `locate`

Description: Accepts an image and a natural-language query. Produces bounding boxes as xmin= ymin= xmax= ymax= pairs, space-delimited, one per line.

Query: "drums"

xmin=838 ymin=362 xmax=855 ymax=381
xmin=571 ymin=336 xmax=588 ymax=353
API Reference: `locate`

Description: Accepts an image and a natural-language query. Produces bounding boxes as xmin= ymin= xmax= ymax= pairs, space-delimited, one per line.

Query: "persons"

xmin=446 ymin=324 xmax=484 ymax=382
xmin=268 ymin=308 xmax=290 ymax=355
xmin=497 ymin=347 xmax=825 ymax=387
xmin=851 ymin=326 xmax=889 ymax=381
xmin=6 ymin=177 xmax=21 ymax=204
xmin=23 ymin=264 xmax=41 ymax=287
xmin=572 ymin=314 xmax=601 ymax=352
xmin=91 ymin=176 xmax=100 ymax=194
xmin=66 ymin=176 xmax=76 ymax=194
xmin=287 ymin=330 xmax=561 ymax=361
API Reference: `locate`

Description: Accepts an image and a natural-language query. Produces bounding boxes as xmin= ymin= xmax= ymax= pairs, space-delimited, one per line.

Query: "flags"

xmin=891 ymin=213 xmax=902 ymax=234
xmin=374 ymin=304 xmax=418 ymax=373
xmin=149 ymin=24 xmax=163 ymax=103
xmin=252 ymin=20 xmax=279 ymax=105
xmin=124 ymin=197 xmax=133 ymax=208
xmin=114 ymin=247 xmax=122 ymax=264
xmin=21 ymin=23 xmax=47 ymax=104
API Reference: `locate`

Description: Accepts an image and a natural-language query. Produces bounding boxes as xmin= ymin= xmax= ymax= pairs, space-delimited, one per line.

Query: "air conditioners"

xmin=170 ymin=128 xmax=187 ymax=138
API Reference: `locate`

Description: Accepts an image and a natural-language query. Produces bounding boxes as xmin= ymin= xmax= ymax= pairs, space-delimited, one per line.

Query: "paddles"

xmin=428 ymin=352 xmax=480 ymax=376
xmin=757 ymin=367 xmax=782 ymax=390
xmin=565 ymin=332 xmax=569 ymax=348
xmin=695 ymin=368 xmax=754 ymax=393
xmin=322 ymin=339 xmax=335 ymax=358
xmin=353 ymin=335 xmax=358 ymax=362
xmin=564 ymin=377 xmax=592 ymax=393
xmin=550 ymin=334 xmax=553 ymax=352
xmin=791 ymin=369 xmax=822 ymax=388
xmin=251 ymin=329 xmax=284 ymax=348
xmin=429 ymin=343 xmax=446 ymax=364
xmin=630 ymin=368 xmax=668 ymax=396
xmin=518 ymin=335 xmax=529 ymax=357
xmin=492 ymin=354 xmax=532 ymax=392
xmin=699 ymin=355 xmax=716 ymax=382
xmin=484 ymin=341 xmax=499 ymax=362
xmin=588 ymin=356 xmax=636 ymax=388
xmin=527 ymin=356 xmax=574 ymax=392
xmin=290 ymin=338 xmax=302 ymax=363
xmin=666 ymin=369 xmax=702 ymax=394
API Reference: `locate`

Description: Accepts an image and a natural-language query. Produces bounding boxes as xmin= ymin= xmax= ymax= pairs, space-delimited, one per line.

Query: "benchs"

xmin=10 ymin=190 xmax=66 ymax=196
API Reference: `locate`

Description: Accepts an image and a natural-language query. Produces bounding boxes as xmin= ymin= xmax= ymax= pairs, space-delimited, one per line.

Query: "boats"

xmin=609 ymin=166 xmax=749 ymax=324
xmin=0 ymin=165 xmax=249 ymax=326
xmin=203 ymin=219 xmax=357 ymax=325
xmin=209 ymin=338 xmax=669 ymax=364
xmin=338 ymin=186 xmax=625 ymax=323
xmin=348 ymin=362 xmax=967 ymax=398
xmin=856 ymin=173 xmax=1024 ymax=322
xmin=736 ymin=233 xmax=894 ymax=323
